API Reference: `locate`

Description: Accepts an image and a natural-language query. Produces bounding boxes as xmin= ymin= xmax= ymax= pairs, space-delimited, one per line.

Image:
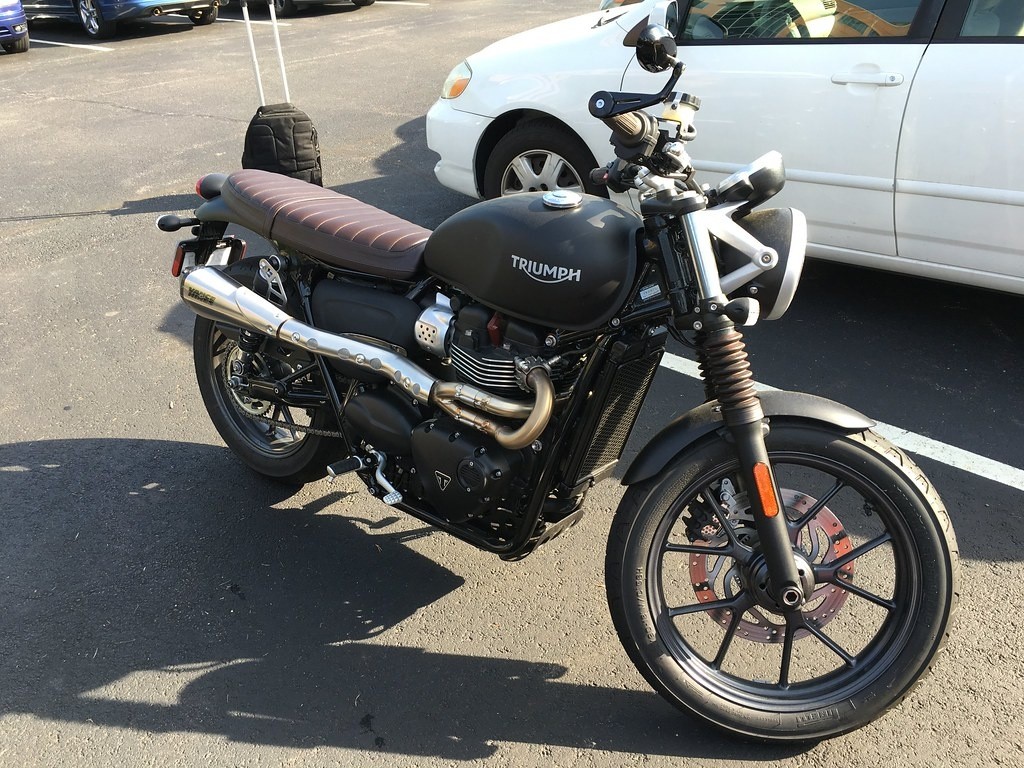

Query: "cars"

xmin=0 ymin=0 xmax=376 ymax=56
xmin=425 ymin=0 xmax=1024 ymax=299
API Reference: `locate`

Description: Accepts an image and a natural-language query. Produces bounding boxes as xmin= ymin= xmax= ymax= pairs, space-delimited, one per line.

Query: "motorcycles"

xmin=156 ymin=25 xmax=962 ymax=749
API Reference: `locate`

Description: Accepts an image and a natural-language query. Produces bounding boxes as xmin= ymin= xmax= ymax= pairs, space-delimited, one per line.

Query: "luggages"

xmin=240 ymin=0 xmax=323 ymax=189
